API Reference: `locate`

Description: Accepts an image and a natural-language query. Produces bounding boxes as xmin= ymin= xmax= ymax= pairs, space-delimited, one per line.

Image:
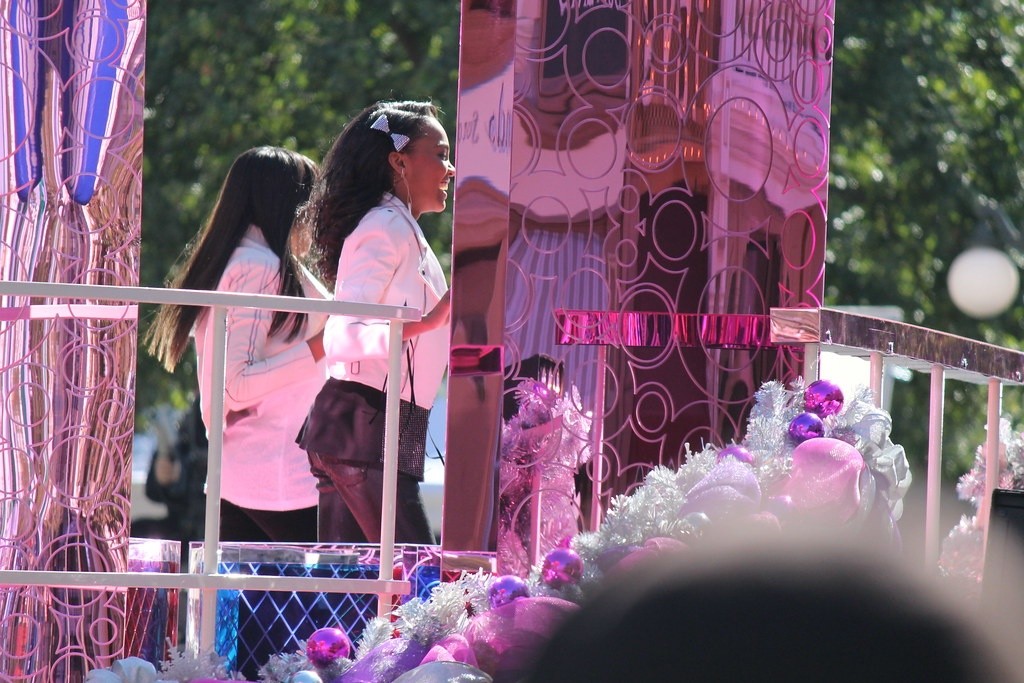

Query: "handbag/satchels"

xmin=294 ymin=340 xmax=430 ymax=480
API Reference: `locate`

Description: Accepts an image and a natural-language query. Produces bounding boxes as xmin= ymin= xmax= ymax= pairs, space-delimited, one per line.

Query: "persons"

xmin=146 ymin=396 xmax=209 ymax=655
xmin=525 ymin=535 xmax=1003 ymax=683
xmin=144 ymin=147 xmax=333 ymax=576
xmin=295 ymin=99 xmax=454 ymax=604
